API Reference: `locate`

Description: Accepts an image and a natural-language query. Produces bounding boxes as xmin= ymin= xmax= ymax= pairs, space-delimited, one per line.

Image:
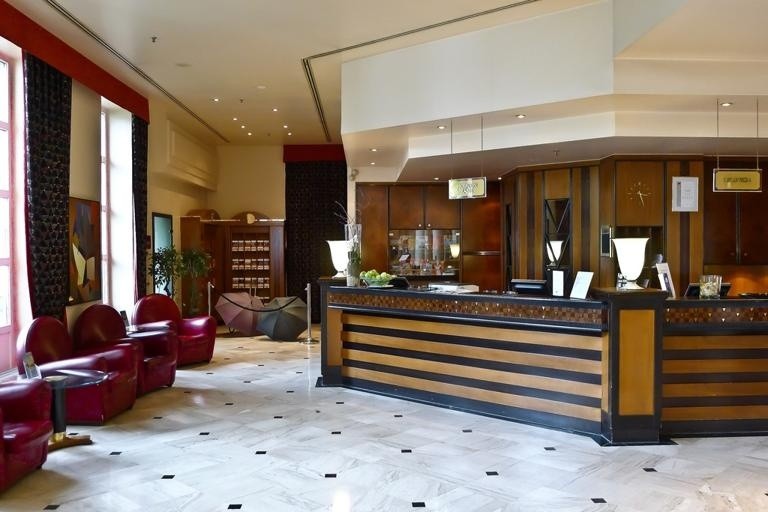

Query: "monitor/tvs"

xmin=230 ymin=238 xmax=271 ymax=290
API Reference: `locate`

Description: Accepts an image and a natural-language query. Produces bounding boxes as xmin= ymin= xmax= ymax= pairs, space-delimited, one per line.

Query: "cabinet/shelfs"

xmin=434 ymin=255 xmax=442 ymax=275
xmin=182 ymin=210 xmax=284 ymax=327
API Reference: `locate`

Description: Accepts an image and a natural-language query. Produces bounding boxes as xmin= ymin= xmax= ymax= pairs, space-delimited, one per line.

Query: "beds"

xmin=213 ymin=291 xmax=265 ymax=338
xmin=256 ymin=294 xmax=307 ymax=341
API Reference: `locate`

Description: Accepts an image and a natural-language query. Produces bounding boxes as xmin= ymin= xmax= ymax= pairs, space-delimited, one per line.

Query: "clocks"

xmin=626 ymin=181 xmax=653 ymax=208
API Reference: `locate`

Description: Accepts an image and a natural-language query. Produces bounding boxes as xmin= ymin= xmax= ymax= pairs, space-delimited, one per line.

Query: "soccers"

xmin=361 ymin=270 xmax=391 ymax=279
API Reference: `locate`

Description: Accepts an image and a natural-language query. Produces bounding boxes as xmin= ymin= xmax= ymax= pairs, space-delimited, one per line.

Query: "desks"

xmin=2 ymin=369 xmax=110 ymax=453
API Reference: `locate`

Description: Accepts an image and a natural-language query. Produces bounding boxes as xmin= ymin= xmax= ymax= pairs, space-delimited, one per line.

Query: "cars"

xmin=700 ymin=274 xmax=722 ymax=300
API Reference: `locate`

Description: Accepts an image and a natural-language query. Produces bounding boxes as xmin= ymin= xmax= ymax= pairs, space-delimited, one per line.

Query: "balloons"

xmin=626 ymin=181 xmax=653 ymax=208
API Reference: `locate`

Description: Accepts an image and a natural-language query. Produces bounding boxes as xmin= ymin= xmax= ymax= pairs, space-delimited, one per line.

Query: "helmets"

xmin=738 ymin=293 xmax=768 ymax=299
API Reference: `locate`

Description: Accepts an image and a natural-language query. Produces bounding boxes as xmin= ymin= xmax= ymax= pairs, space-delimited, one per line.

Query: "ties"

xmin=511 ymin=279 xmax=551 ymax=297
xmin=390 ymin=277 xmax=410 ymax=288
xmin=684 ymin=283 xmax=732 ymax=296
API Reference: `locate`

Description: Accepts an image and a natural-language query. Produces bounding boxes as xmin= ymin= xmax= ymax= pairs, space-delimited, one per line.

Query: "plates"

xmin=182 ymin=210 xmax=284 ymax=327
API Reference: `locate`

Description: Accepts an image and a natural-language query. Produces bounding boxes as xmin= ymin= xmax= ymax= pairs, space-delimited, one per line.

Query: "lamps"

xmin=326 ymin=240 xmax=355 ymax=280
xmin=543 ymin=237 xmax=567 ymax=283
xmin=610 ymin=235 xmax=649 ymax=292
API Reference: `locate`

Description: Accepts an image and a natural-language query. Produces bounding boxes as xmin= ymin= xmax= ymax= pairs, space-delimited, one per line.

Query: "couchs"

xmin=131 ymin=294 xmax=216 ymax=367
xmin=71 ymin=304 xmax=178 ymax=398
xmin=0 ymin=380 xmax=53 ymax=493
xmin=16 ymin=316 xmax=140 ymax=427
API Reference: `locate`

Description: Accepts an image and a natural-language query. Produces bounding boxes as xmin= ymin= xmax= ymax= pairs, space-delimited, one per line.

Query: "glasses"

xmin=610 ymin=235 xmax=649 ymax=292
xmin=543 ymin=237 xmax=567 ymax=283
xmin=326 ymin=240 xmax=355 ymax=280
